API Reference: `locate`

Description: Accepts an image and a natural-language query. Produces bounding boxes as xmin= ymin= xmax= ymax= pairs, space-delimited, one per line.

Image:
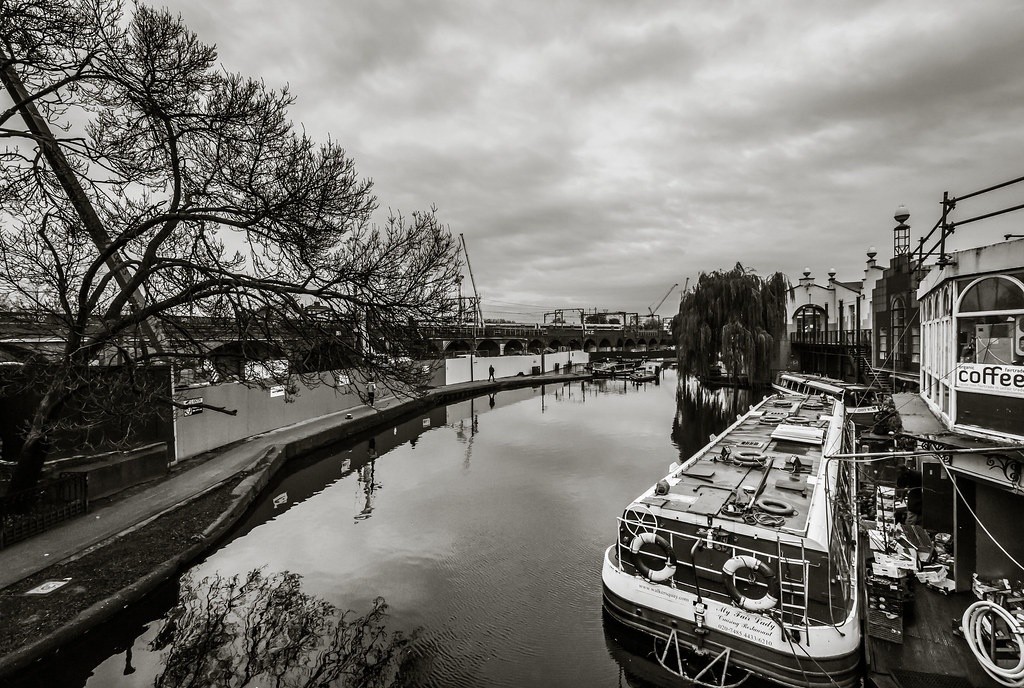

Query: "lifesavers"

xmin=759 ymin=416 xmax=783 ymax=427
xmin=786 ymin=416 xmax=810 ymax=424
xmin=629 ymin=532 xmax=677 ymax=582
xmin=721 ymin=555 xmax=780 ymax=610
xmin=773 ymin=400 xmax=793 ymax=408
xmin=733 ymin=451 xmax=767 ymax=466
xmin=802 ymin=402 xmax=823 ymax=410
xmin=756 ymin=498 xmax=794 ymax=517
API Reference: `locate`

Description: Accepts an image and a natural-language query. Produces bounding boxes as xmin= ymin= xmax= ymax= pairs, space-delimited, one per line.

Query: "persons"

xmin=364 ymin=379 xmax=376 ymax=409
xmin=488 ymin=366 xmax=497 ymax=382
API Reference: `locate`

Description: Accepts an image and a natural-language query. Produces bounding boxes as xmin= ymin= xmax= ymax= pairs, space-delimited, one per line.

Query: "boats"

xmin=771 ymin=368 xmax=885 ymax=422
xmin=601 ymin=386 xmax=872 ymax=688
xmin=587 ymin=355 xmax=666 ymax=382
